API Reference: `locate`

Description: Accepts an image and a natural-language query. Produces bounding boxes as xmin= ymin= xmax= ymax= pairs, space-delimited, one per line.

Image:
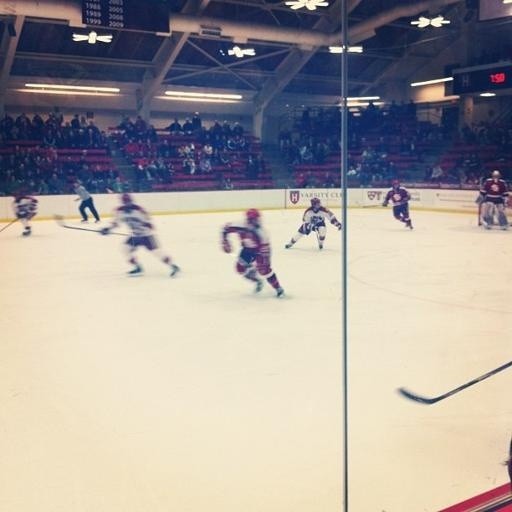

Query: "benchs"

xmin=439 ymin=123 xmax=511 ymax=185
xmin=0 ymin=111 xmax=129 ymax=194
xmin=281 ymin=113 xmax=437 ymax=186
xmin=109 ymin=127 xmax=274 ymax=192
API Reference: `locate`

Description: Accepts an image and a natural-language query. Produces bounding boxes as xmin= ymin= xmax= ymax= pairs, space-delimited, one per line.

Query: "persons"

xmin=2 ymin=106 xmax=132 ymax=195
xmin=72 ymin=178 xmax=100 ymax=224
xmin=423 ymin=112 xmax=512 ymax=185
xmin=220 ymin=207 xmax=285 ymax=299
xmin=166 ymin=112 xmax=266 ymax=178
xmin=284 ymin=198 xmax=343 ymax=252
xmin=11 ymin=186 xmax=39 ymax=237
xmin=109 ymin=115 xmax=178 ymax=189
xmin=479 ymin=170 xmax=510 ymax=231
xmin=99 ymin=191 xmax=182 ymax=279
xmin=277 ymin=99 xmax=432 ymax=188
xmin=383 ymin=180 xmax=416 ymax=232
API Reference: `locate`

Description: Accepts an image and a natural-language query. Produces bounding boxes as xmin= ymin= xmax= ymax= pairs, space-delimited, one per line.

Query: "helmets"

xmin=311 ymin=198 xmax=320 ymax=205
xmin=246 ymin=208 xmax=260 ymax=219
xmin=392 ymin=180 xmax=400 ymax=187
xmin=122 ymin=194 xmax=130 ymax=202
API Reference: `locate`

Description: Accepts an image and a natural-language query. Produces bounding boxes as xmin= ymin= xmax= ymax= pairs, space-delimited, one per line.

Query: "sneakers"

xmin=405 ymin=223 xmax=413 ymax=230
xmin=22 ymin=229 xmax=31 ymax=235
xmin=95 ymin=219 xmax=101 ymax=223
xmin=128 ymin=265 xmax=144 ymax=277
xmin=285 ymin=243 xmax=293 ymax=248
xmin=81 ymin=219 xmax=89 ymax=224
xmin=318 ymin=244 xmax=324 ymax=249
xmin=255 ymin=279 xmax=284 ymax=298
xmin=168 ymin=264 xmax=180 ymax=277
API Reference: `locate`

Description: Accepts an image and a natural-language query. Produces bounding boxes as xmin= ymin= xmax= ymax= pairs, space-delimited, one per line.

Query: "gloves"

xmin=335 ymin=222 xmax=341 ymax=231
xmin=101 ymin=228 xmax=109 ymax=235
xmin=223 ymin=240 xmax=232 ymax=253
